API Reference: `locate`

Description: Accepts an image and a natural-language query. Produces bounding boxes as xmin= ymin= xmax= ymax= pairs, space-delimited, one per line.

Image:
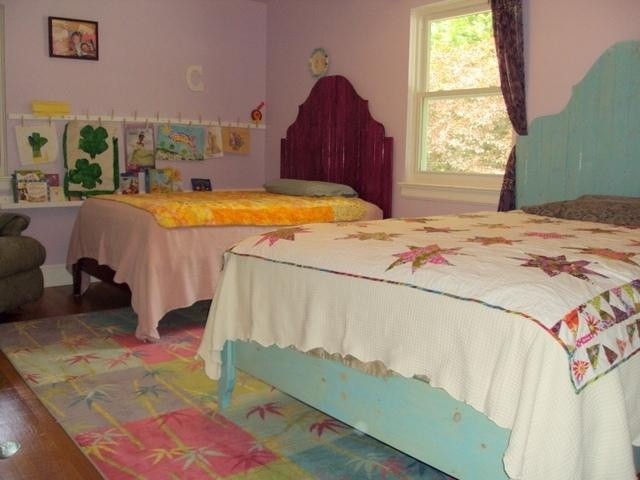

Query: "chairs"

xmin=0 ymin=211 xmax=46 ymax=313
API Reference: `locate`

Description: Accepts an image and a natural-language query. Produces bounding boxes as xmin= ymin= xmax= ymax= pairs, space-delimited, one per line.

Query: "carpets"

xmin=1 ymin=307 xmax=458 ymax=480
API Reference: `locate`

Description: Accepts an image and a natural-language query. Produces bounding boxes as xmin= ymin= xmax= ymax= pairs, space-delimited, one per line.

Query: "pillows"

xmin=263 ymin=178 xmax=358 ymax=198
xmin=520 ymin=194 xmax=640 ymax=227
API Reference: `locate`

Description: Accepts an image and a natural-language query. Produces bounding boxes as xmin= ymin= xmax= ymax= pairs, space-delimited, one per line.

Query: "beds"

xmin=217 ymin=39 xmax=640 ymax=480
xmin=73 ymin=75 xmax=394 ymax=339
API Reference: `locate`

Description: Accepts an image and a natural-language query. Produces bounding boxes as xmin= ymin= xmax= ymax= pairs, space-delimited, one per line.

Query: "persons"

xmin=128 ymin=131 xmax=153 ymax=166
xmin=79 ymin=42 xmax=97 ymax=58
xmin=71 ymin=31 xmax=94 ymax=57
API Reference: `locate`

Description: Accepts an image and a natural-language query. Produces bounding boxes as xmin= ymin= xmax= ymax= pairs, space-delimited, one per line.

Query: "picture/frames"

xmin=48 ymin=15 xmax=98 ymax=60
